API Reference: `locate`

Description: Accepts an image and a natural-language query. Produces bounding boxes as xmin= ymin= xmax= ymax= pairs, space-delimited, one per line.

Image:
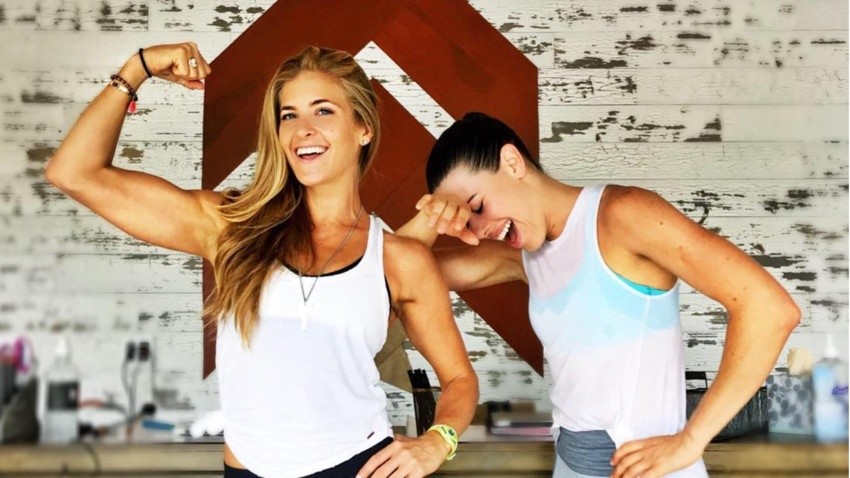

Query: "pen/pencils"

xmin=406 ymin=369 xmax=436 ymax=436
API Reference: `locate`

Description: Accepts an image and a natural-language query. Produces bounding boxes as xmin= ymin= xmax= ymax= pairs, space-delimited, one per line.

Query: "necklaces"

xmin=297 ymin=206 xmax=360 ymax=304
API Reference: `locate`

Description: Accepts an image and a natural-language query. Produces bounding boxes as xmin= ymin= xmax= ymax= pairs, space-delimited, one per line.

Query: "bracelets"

xmin=139 ymin=49 xmax=154 ymax=78
xmin=108 ymin=75 xmax=139 ymax=113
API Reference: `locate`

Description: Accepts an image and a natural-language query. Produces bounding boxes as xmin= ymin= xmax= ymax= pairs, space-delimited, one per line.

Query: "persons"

xmin=45 ymin=42 xmax=479 ymax=478
xmin=388 ymin=111 xmax=802 ymax=478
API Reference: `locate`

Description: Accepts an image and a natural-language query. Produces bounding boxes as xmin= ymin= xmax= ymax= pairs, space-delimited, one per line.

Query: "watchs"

xmin=426 ymin=424 xmax=459 ymax=461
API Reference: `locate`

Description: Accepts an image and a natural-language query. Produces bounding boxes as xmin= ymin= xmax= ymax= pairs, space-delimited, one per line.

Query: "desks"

xmin=0 ymin=433 xmax=849 ymax=478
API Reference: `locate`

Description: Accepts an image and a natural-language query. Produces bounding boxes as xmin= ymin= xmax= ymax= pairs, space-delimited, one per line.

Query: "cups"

xmin=152 ymin=337 xmax=195 ymax=421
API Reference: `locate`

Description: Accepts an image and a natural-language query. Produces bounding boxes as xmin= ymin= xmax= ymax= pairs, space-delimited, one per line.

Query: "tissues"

xmin=765 ymin=346 xmax=816 ymax=435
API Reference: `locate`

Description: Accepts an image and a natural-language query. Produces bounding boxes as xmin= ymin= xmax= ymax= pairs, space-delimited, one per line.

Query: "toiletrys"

xmin=43 ymin=336 xmax=82 ymax=446
xmin=813 ymin=334 xmax=848 ymax=443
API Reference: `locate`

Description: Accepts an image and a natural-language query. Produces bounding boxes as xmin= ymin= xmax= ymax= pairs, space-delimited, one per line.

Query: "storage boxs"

xmin=686 ymin=385 xmax=775 ymax=444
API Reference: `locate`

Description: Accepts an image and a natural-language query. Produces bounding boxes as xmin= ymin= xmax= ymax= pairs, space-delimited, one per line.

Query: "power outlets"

xmin=124 ymin=332 xmax=154 ymax=371
